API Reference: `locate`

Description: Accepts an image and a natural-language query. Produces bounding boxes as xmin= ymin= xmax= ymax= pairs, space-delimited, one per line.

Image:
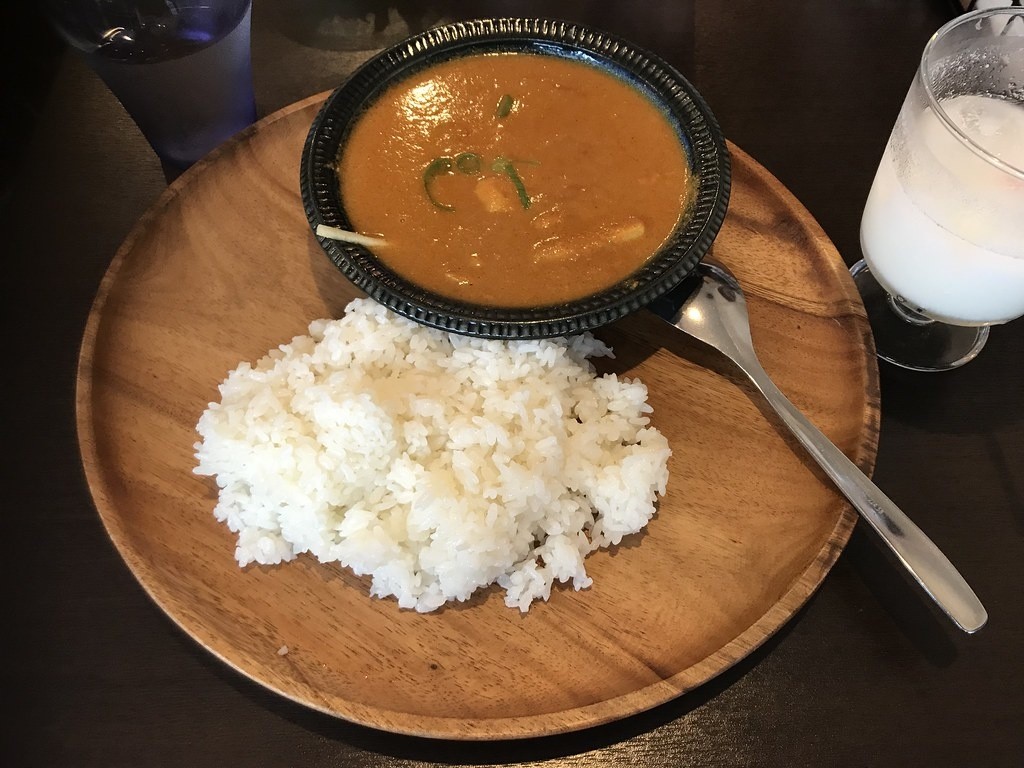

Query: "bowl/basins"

xmin=299 ymin=15 xmax=732 ymax=341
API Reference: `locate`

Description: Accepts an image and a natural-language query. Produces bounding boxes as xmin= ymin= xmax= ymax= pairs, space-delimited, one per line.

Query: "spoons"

xmin=645 ymin=253 xmax=987 ymax=635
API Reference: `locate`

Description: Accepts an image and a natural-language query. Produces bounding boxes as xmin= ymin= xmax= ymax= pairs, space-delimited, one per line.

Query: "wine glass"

xmin=846 ymin=7 xmax=1024 ymax=372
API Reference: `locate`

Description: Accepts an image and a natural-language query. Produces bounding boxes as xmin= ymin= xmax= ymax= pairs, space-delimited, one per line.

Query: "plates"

xmin=75 ymin=85 xmax=882 ymax=739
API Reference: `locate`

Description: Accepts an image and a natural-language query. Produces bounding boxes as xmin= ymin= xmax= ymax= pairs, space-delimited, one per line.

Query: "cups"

xmin=55 ymin=0 xmax=258 ymax=172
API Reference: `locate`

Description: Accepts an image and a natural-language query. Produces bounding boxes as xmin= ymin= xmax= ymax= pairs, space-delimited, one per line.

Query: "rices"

xmin=190 ymin=297 xmax=672 ymax=615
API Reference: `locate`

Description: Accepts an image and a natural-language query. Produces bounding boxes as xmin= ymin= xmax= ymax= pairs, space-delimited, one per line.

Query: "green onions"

xmin=424 ymin=93 xmax=533 ymax=211
